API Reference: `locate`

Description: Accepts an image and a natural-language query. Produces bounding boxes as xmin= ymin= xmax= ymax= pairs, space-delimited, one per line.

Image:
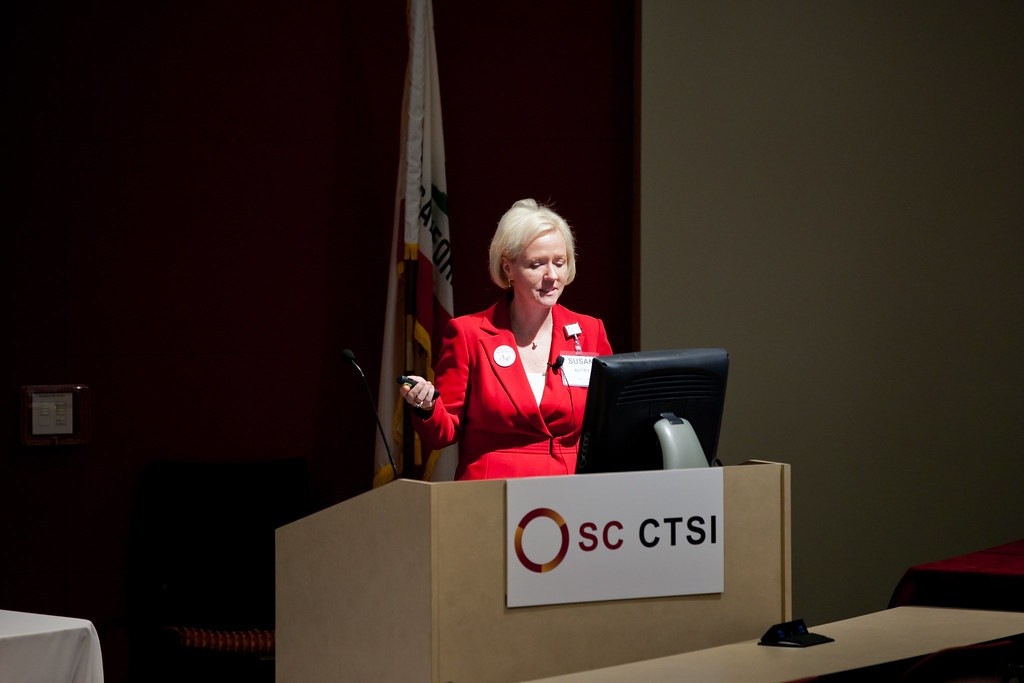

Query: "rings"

xmin=414 ymin=402 xmax=423 ymax=408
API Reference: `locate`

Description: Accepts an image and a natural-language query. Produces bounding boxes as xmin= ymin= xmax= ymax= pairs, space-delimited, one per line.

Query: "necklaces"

xmin=511 ymin=323 xmax=552 ymax=348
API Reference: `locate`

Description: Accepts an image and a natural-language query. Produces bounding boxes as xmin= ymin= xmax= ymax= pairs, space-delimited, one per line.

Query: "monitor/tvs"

xmin=575 ymin=346 xmax=729 ymax=475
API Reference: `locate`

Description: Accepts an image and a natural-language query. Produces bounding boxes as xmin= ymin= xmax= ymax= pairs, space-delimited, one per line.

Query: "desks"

xmin=0 ymin=609 xmax=104 ymax=683
xmin=521 ymin=539 xmax=1024 ymax=683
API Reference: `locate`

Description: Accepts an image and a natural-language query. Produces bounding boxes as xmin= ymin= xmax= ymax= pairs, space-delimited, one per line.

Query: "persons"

xmin=399 ymin=199 xmax=613 ymax=484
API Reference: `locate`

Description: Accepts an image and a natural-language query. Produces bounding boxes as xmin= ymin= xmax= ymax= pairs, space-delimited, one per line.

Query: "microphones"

xmin=551 ymin=356 xmax=564 ymax=369
xmin=342 ymin=348 xmax=398 ymax=480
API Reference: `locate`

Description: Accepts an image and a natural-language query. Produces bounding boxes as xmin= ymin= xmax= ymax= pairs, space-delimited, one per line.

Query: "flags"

xmin=372 ymin=0 xmax=458 ymax=489
